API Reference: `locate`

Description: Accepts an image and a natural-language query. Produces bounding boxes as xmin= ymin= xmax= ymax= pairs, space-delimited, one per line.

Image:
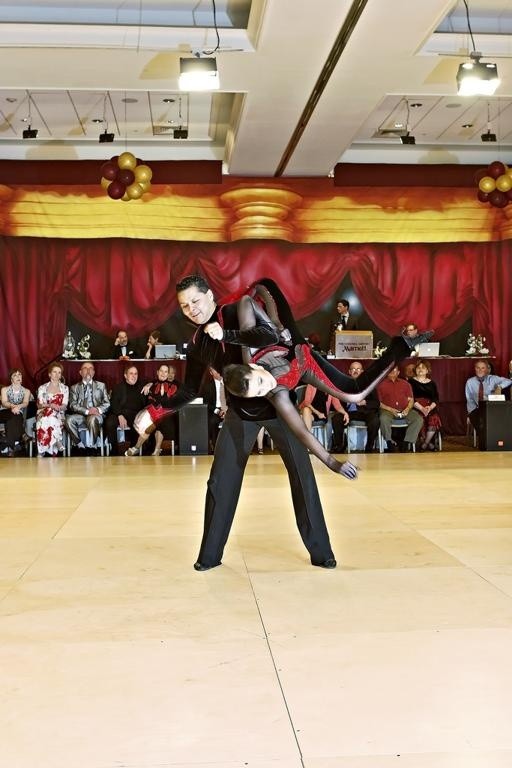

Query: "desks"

xmin=59 ymin=354 xmax=494 ymax=438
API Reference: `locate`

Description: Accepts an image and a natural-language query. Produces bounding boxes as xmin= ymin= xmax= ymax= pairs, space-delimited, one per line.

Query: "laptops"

xmin=417 ymin=342 xmax=440 ymax=358
xmin=155 ymin=345 xmax=176 ymax=358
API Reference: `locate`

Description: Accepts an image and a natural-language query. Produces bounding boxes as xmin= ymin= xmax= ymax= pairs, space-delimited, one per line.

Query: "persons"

xmin=399 ymin=323 xmax=435 ymax=356
xmin=1 ymin=366 xmax=33 ymax=458
xmin=329 ymin=299 xmax=358 ymax=354
xmin=34 ymin=360 xmax=70 ymax=457
xmin=220 ymin=294 xmax=435 ymax=480
xmin=64 ymin=361 xmax=112 ymax=456
xmin=122 ymin=359 xmax=264 ymax=455
xmin=463 ymin=359 xmax=512 ymax=451
xmin=110 ymin=329 xmax=139 ymax=359
xmin=143 ymin=330 xmax=165 ymax=359
xmin=288 ymin=360 xmax=443 ymax=455
xmin=104 ymin=365 xmax=152 ymax=454
xmin=132 ymin=274 xmax=337 ymax=570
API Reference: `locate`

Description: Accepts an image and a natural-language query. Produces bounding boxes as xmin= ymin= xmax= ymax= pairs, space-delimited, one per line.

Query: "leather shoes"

xmin=7 ymin=433 xmax=162 ymax=456
xmin=194 ymin=561 xmax=222 ymax=571
xmin=366 ymin=437 xmax=439 ymax=452
xmin=312 ymin=559 xmax=336 ymax=569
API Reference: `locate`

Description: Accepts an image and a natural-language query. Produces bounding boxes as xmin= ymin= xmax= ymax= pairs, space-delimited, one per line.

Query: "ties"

xmin=479 ymin=379 xmax=484 ymax=400
xmin=342 ymin=316 xmax=346 ymax=329
xmin=85 ymin=383 xmax=93 ymax=408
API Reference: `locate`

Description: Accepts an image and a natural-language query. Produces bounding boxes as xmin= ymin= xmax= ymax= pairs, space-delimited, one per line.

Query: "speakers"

xmin=179 ymin=404 xmax=208 ymax=455
xmin=479 ymin=400 xmax=512 ymax=451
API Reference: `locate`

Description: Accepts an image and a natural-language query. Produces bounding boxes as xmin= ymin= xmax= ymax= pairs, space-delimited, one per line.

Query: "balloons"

xmin=100 ymin=152 xmax=153 ymax=202
xmin=474 ymin=161 xmax=511 ymax=209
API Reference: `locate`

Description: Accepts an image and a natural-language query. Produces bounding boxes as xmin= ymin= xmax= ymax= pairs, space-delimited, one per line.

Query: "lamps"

xmin=99 ymin=93 xmax=115 ymax=142
xmin=454 ymin=1 xmax=500 ymax=96
xmin=23 ymin=96 xmax=39 ymax=139
xmin=480 ymin=102 xmax=498 ymax=140
xmin=173 ymin=98 xmax=188 ymax=140
xmin=177 ymin=0 xmax=222 ymax=91
xmin=399 ymin=99 xmax=417 ymax=145
